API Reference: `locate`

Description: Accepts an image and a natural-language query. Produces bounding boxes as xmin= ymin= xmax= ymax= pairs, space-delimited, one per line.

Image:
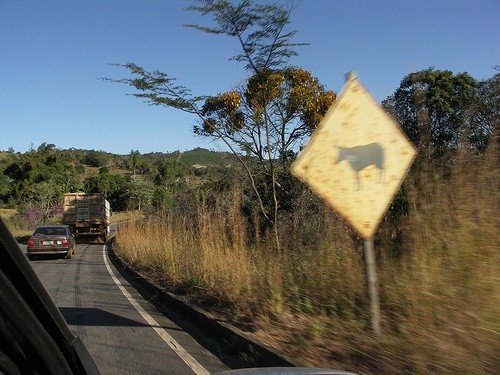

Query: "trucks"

xmin=62 ymin=191 xmax=112 ymax=244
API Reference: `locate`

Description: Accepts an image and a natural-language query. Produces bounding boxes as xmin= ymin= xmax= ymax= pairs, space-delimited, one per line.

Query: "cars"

xmin=27 ymin=226 xmax=76 ymax=260
